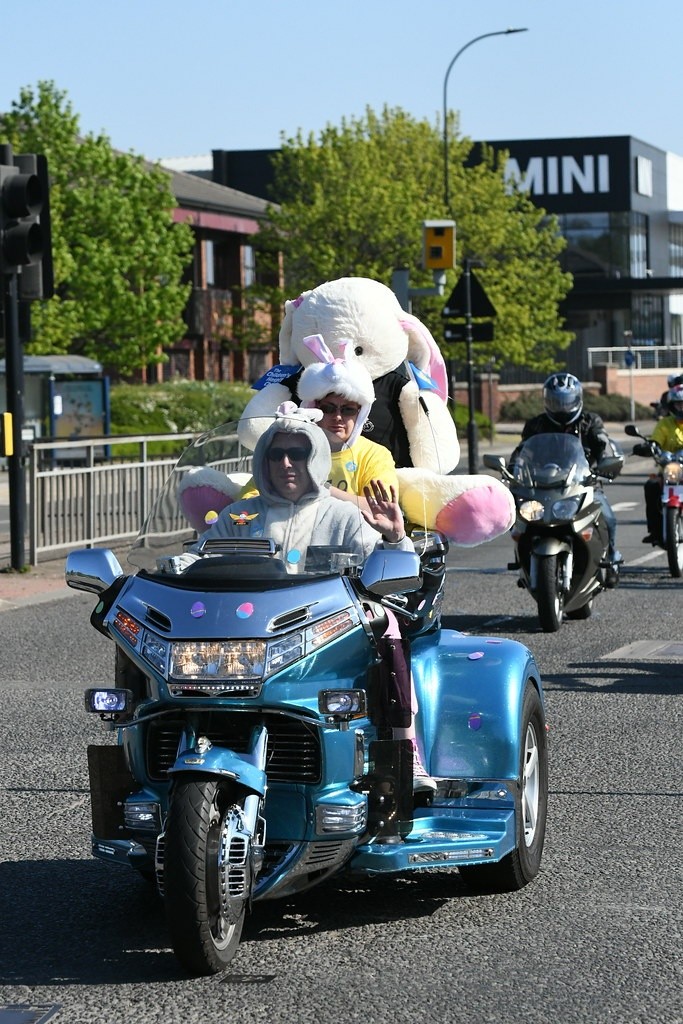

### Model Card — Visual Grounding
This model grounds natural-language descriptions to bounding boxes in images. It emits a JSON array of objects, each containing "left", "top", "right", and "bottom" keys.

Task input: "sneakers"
[
  {"left": 640, "top": 531, "right": 661, "bottom": 543},
  {"left": 604, "top": 563, "right": 621, "bottom": 587},
  {"left": 394, "top": 735, "right": 438, "bottom": 793}
]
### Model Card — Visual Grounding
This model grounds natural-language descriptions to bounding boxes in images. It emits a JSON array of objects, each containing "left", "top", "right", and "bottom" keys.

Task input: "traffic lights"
[{"left": 0, "top": 164, "right": 43, "bottom": 278}]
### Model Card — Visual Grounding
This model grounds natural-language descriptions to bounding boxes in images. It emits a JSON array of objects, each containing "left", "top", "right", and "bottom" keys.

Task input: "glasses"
[
  {"left": 266, "top": 446, "right": 308, "bottom": 461},
  {"left": 318, "top": 401, "right": 361, "bottom": 416}
]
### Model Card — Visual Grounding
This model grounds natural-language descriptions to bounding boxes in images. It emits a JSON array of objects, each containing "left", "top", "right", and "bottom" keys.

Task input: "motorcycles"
[
  {"left": 58, "top": 415, "right": 552, "bottom": 980},
  {"left": 624, "top": 422, "right": 683, "bottom": 579},
  {"left": 483, "top": 432, "right": 625, "bottom": 633}
]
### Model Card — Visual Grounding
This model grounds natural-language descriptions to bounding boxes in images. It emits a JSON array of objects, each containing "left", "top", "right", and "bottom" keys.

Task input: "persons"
[
  {"left": 229, "top": 362, "right": 400, "bottom": 519},
  {"left": 631, "top": 385, "right": 683, "bottom": 545},
  {"left": 183, "top": 401, "right": 438, "bottom": 805},
  {"left": 507, "top": 372, "right": 625, "bottom": 592}
]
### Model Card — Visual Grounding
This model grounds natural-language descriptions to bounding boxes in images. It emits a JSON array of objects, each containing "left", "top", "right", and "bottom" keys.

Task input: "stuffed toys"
[{"left": 177, "top": 277, "right": 517, "bottom": 551}]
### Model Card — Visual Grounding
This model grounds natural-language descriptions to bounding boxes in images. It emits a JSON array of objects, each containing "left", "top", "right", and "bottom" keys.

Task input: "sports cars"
[{"left": 649, "top": 400, "right": 672, "bottom": 422}]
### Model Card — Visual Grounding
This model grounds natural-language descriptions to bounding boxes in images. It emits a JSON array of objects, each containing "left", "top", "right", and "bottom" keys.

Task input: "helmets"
[
  {"left": 666, "top": 371, "right": 679, "bottom": 385},
  {"left": 668, "top": 384, "right": 683, "bottom": 420},
  {"left": 543, "top": 371, "right": 583, "bottom": 426}
]
